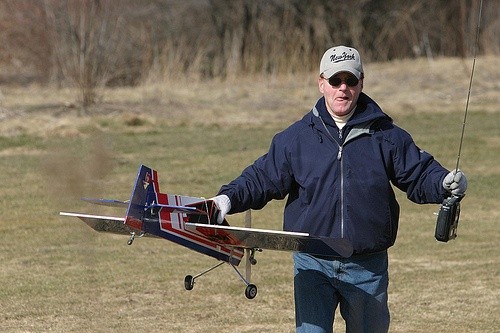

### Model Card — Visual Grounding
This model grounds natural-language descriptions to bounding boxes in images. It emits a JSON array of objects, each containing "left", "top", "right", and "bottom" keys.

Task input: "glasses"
[{"left": 319, "top": 75, "right": 364, "bottom": 85}]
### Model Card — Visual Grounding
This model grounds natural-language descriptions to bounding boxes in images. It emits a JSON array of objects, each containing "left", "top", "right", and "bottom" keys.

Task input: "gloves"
[
  {"left": 206, "top": 194, "right": 231, "bottom": 224},
  {"left": 442, "top": 168, "right": 467, "bottom": 196}
]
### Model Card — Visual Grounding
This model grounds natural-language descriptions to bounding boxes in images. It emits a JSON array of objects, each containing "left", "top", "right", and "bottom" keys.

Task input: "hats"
[{"left": 319, "top": 45, "right": 364, "bottom": 80}]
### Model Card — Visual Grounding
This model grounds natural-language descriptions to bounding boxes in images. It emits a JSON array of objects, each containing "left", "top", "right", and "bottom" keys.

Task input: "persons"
[{"left": 204, "top": 42, "right": 469, "bottom": 333}]
